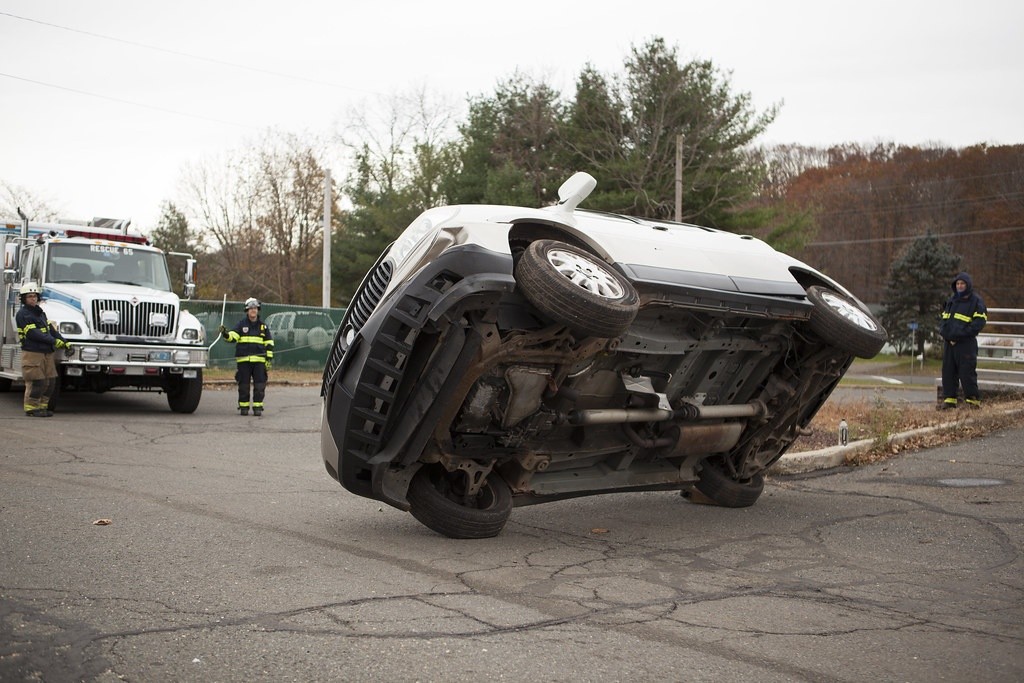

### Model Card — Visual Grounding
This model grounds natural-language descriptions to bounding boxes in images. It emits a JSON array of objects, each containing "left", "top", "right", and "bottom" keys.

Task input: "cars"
[{"left": 320, "top": 171, "right": 888, "bottom": 539}]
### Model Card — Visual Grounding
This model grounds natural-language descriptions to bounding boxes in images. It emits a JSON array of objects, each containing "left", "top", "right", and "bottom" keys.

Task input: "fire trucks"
[{"left": 0, "top": 207, "right": 227, "bottom": 414}]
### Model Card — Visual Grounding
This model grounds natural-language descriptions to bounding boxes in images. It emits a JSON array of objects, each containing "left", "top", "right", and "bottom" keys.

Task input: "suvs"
[{"left": 188, "top": 311, "right": 340, "bottom": 368}]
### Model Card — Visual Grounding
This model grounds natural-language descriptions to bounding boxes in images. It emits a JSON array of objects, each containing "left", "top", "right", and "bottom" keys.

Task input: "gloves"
[
  {"left": 218, "top": 325, "right": 227, "bottom": 337},
  {"left": 53, "top": 338, "right": 66, "bottom": 350},
  {"left": 265, "top": 361, "right": 273, "bottom": 371},
  {"left": 64, "top": 342, "right": 71, "bottom": 350}
]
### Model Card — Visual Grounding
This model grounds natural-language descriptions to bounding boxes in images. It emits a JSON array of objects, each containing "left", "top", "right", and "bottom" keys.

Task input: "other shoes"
[
  {"left": 254, "top": 408, "right": 261, "bottom": 416},
  {"left": 26, "top": 408, "right": 54, "bottom": 417},
  {"left": 241, "top": 407, "right": 248, "bottom": 415},
  {"left": 966, "top": 402, "right": 980, "bottom": 410},
  {"left": 940, "top": 403, "right": 956, "bottom": 411}
]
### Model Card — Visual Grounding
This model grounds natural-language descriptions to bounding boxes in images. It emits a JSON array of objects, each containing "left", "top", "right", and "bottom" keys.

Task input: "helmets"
[
  {"left": 19, "top": 282, "right": 39, "bottom": 295},
  {"left": 244, "top": 297, "right": 260, "bottom": 311}
]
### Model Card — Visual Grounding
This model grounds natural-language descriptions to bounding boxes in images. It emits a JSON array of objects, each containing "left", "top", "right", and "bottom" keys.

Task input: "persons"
[
  {"left": 219, "top": 297, "right": 274, "bottom": 415},
  {"left": 15, "top": 282, "right": 71, "bottom": 416},
  {"left": 936, "top": 272, "right": 987, "bottom": 410}
]
